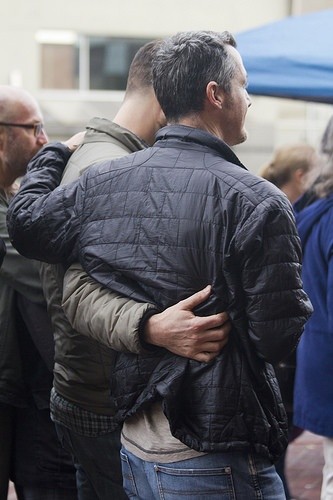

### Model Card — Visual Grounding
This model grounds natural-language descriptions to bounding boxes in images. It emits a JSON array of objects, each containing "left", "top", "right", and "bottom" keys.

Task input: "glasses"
[{"left": 1, "top": 121, "right": 45, "bottom": 137}]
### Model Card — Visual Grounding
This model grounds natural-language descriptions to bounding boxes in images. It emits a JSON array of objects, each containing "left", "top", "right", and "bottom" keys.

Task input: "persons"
[
  {"left": 0, "top": 84, "right": 82, "bottom": 499},
  {"left": 7, "top": 30, "right": 314, "bottom": 499},
  {"left": 258, "top": 141, "right": 321, "bottom": 208},
  {"left": 291, "top": 112, "right": 333, "bottom": 500},
  {"left": 34, "top": 36, "right": 230, "bottom": 498}
]
